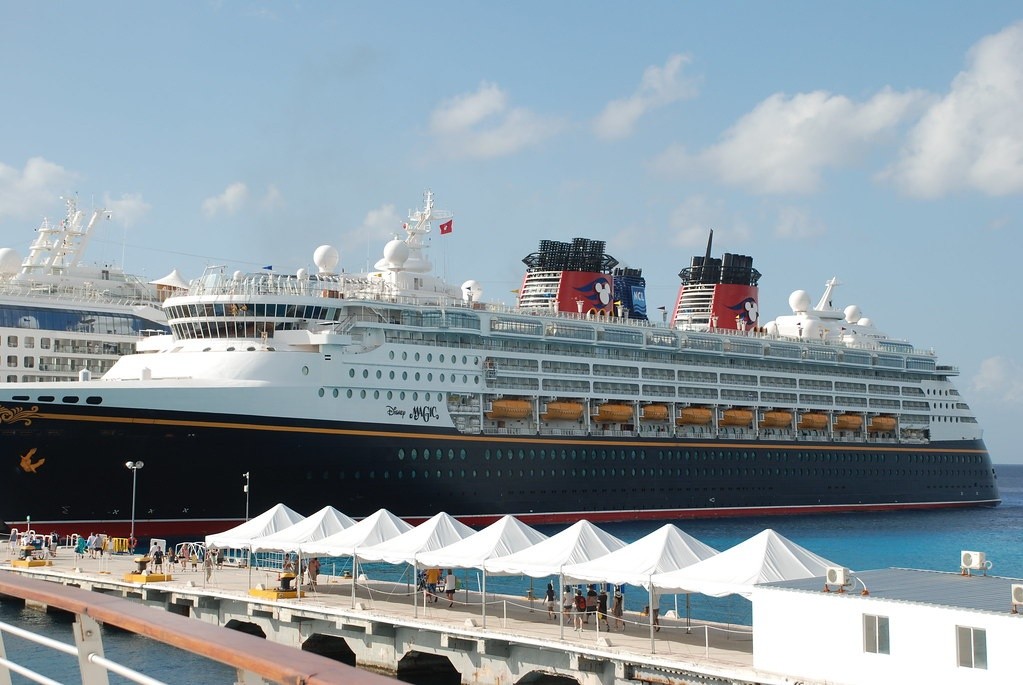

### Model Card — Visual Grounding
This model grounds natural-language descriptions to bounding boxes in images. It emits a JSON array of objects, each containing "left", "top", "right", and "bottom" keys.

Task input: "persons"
[
  {"left": 443, "top": 569, "right": 455, "bottom": 608},
  {"left": 148, "top": 542, "right": 223, "bottom": 583},
  {"left": 26, "top": 530, "right": 30, "bottom": 542},
  {"left": 75, "top": 535, "right": 86, "bottom": 559},
  {"left": 389, "top": 315, "right": 395, "bottom": 324},
  {"left": 107, "top": 536, "right": 114, "bottom": 560},
  {"left": 542, "top": 583, "right": 661, "bottom": 632},
  {"left": 657, "top": 426, "right": 660, "bottom": 432},
  {"left": 283, "top": 552, "right": 293, "bottom": 572},
  {"left": 426, "top": 569, "right": 441, "bottom": 603},
  {"left": 29, "top": 535, "right": 34, "bottom": 545},
  {"left": 293, "top": 554, "right": 304, "bottom": 589},
  {"left": 87, "top": 532, "right": 103, "bottom": 559},
  {"left": 8, "top": 530, "right": 18, "bottom": 555},
  {"left": 128, "top": 533, "right": 137, "bottom": 555},
  {"left": 48, "top": 530, "right": 59, "bottom": 557},
  {"left": 94, "top": 344, "right": 100, "bottom": 354},
  {"left": 307, "top": 557, "right": 321, "bottom": 586}
]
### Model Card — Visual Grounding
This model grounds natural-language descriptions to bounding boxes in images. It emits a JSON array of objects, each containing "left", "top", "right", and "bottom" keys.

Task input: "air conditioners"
[
  {"left": 826, "top": 567, "right": 851, "bottom": 586},
  {"left": 1011, "top": 584, "right": 1023, "bottom": 606},
  {"left": 960, "top": 551, "right": 986, "bottom": 570}
]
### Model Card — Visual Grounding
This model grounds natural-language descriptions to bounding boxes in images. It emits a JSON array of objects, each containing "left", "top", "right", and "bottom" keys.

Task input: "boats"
[
  {"left": 797, "top": 413, "right": 828, "bottom": 429},
  {"left": 759, "top": 410, "right": 793, "bottom": 429},
  {"left": 868, "top": 417, "right": 897, "bottom": 432},
  {"left": 484, "top": 399, "right": 533, "bottom": 421},
  {"left": 675, "top": 408, "right": 713, "bottom": 425},
  {"left": 640, "top": 403, "right": 672, "bottom": 422},
  {"left": 833, "top": 414, "right": 863, "bottom": 429},
  {"left": 719, "top": 408, "right": 757, "bottom": 427},
  {"left": 539, "top": 401, "right": 585, "bottom": 423},
  {"left": 591, "top": 404, "right": 634, "bottom": 422}
]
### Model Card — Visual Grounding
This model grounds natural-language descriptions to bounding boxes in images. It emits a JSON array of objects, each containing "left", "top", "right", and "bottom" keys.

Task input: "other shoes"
[
  {"left": 656, "top": 627, "right": 661, "bottom": 632},
  {"left": 566, "top": 618, "right": 571, "bottom": 623},
  {"left": 435, "top": 597, "right": 437, "bottom": 601},
  {"left": 553, "top": 615, "right": 556, "bottom": 620},
  {"left": 427, "top": 600, "right": 432, "bottom": 603},
  {"left": 449, "top": 602, "right": 454, "bottom": 607}
]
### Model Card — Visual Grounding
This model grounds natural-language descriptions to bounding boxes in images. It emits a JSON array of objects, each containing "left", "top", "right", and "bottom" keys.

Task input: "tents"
[
  {"left": 296, "top": 508, "right": 417, "bottom": 602},
  {"left": 649, "top": 529, "right": 856, "bottom": 655},
  {"left": 351, "top": 512, "right": 479, "bottom": 612},
  {"left": 481, "top": 520, "right": 630, "bottom": 630},
  {"left": 248, "top": 506, "right": 358, "bottom": 590},
  {"left": 559, "top": 523, "right": 722, "bottom": 641},
  {"left": 414, "top": 513, "right": 551, "bottom": 619},
  {"left": 204, "top": 503, "right": 307, "bottom": 586}
]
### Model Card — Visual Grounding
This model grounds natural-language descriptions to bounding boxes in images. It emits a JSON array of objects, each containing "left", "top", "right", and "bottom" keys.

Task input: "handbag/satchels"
[
  {"left": 73, "top": 545, "right": 80, "bottom": 552},
  {"left": 598, "top": 613, "right": 603, "bottom": 620},
  {"left": 456, "top": 579, "right": 462, "bottom": 589}
]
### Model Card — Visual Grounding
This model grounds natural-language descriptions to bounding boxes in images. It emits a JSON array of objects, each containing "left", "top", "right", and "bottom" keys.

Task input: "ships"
[
  {"left": 1, "top": 193, "right": 1003, "bottom": 543},
  {"left": 2, "top": 197, "right": 186, "bottom": 382}
]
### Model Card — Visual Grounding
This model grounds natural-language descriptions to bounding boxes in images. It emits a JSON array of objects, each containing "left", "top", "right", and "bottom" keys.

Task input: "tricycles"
[{"left": 416, "top": 570, "right": 446, "bottom": 593}]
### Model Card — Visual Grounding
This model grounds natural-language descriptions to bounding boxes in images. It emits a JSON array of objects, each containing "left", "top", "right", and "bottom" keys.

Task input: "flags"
[{"left": 439, "top": 219, "right": 452, "bottom": 234}]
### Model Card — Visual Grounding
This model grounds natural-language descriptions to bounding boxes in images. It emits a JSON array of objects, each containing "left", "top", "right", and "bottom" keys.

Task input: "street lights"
[
  {"left": 242, "top": 471, "right": 252, "bottom": 522},
  {"left": 124, "top": 461, "right": 145, "bottom": 554}
]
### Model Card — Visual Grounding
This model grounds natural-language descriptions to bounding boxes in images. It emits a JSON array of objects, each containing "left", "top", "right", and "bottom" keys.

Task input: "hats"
[
  {"left": 615, "top": 591, "right": 622, "bottom": 598},
  {"left": 600, "top": 589, "right": 605, "bottom": 595}
]
